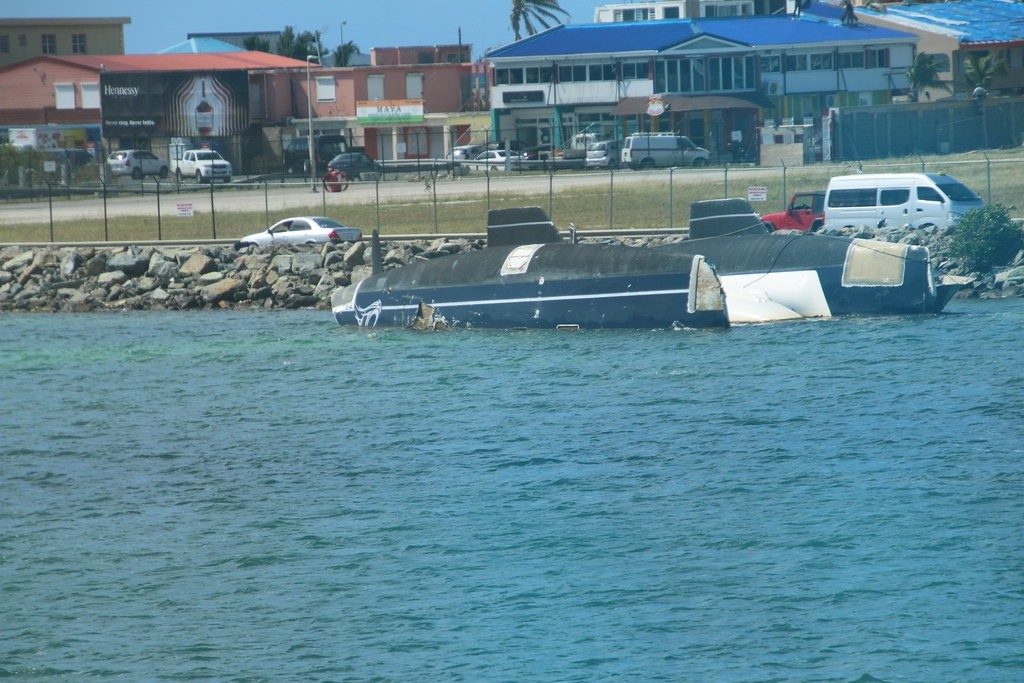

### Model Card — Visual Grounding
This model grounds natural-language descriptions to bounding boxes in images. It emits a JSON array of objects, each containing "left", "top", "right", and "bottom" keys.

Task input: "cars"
[
  {"left": 326, "top": 152, "right": 384, "bottom": 178},
  {"left": 238, "top": 216, "right": 362, "bottom": 248},
  {"left": 473, "top": 150, "right": 523, "bottom": 171}
]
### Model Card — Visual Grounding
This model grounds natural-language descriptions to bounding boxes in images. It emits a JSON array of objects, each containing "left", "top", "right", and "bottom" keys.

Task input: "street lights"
[
  {"left": 340, "top": 21, "right": 346, "bottom": 66},
  {"left": 306, "top": 55, "right": 318, "bottom": 192}
]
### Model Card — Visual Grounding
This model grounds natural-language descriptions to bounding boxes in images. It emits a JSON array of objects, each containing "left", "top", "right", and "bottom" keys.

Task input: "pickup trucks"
[{"left": 172, "top": 149, "right": 232, "bottom": 184}]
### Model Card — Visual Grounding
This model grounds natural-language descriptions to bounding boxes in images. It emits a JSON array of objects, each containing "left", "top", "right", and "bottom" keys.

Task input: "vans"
[
  {"left": 571, "top": 133, "right": 604, "bottom": 149},
  {"left": 282, "top": 134, "right": 348, "bottom": 173},
  {"left": 621, "top": 130, "right": 711, "bottom": 170},
  {"left": 585, "top": 140, "right": 624, "bottom": 168},
  {"left": 447, "top": 146, "right": 480, "bottom": 160},
  {"left": 823, "top": 172, "right": 985, "bottom": 232}
]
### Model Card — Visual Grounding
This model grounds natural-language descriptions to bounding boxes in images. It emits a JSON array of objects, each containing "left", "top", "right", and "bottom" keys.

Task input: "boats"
[{"left": 332, "top": 197, "right": 978, "bottom": 332}]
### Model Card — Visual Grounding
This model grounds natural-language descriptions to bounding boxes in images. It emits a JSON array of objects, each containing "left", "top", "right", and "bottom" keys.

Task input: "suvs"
[
  {"left": 40, "top": 149, "right": 96, "bottom": 181},
  {"left": 759, "top": 190, "right": 827, "bottom": 233},
  {"left": 108, "top": 149, "right": 168, "bottom": 179}
]
